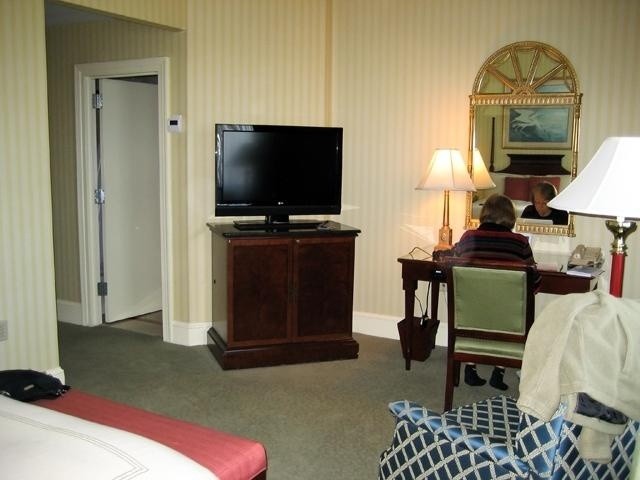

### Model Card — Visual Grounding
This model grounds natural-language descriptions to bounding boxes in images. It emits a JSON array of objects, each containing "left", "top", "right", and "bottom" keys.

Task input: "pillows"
[
  {"left": 529, "top": 177, "right": 559, "bottom": 203},
  {"left": 505, "top": 177, "right": 529, "bottom": 201}
]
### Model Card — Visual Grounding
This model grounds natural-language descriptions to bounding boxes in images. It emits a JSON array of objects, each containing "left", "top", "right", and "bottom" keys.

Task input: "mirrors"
[{"left": 464, "top": 40, "right": 581, "bottom": 237}]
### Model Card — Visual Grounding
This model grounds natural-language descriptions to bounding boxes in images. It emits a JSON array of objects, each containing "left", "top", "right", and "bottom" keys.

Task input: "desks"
[{"left": 397, "top": 244, "right": 605, "bottom": 371}]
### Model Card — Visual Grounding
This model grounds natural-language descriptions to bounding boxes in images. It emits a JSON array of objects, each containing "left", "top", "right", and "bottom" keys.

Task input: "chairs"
[
  {"left": 378, "top": 293, "right": 639, "bottom": 480},
  {"left": 440, "top": 255, "right": 534, "bottom": 411}
]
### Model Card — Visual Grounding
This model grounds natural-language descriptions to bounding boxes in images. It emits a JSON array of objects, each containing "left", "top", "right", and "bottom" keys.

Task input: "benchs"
[{"left": 0, "top": 380, "right": 268, "bottom": 479}]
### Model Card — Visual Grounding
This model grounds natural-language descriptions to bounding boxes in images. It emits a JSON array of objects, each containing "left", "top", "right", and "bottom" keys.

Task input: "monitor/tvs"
[{"left": 215, "top": 124, "right": 343, "bottom": 228}]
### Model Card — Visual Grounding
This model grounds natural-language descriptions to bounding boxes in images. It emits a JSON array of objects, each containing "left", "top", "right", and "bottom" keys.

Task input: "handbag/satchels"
[{"left": 0, "top": 369, "right": 72, "bottom": 403}]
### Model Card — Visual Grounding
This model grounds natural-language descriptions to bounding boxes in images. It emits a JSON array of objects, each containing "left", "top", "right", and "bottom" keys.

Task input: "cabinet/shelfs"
[{"left": 205, "top": 219, "right": 362, "bottom": 370}]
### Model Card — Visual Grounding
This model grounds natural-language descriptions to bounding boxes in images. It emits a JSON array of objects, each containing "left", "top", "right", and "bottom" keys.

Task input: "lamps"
[
  {"left": 414, "top": 148, "right": 477, "bottom": 261},
  {"left": 545, "top": 137, "right": 640, "bottom": 300},
  {"left": 472, "top": 147, "right": 495, "bottom": 190}
]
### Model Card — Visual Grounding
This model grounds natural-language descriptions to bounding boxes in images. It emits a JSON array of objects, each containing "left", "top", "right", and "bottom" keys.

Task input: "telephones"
[{"left": 567, "top": 244, "right": 602, "bottom": 266}]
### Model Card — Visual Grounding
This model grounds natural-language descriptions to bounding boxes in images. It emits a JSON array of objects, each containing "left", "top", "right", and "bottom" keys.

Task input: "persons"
[
  {"left": 451, "top": 192, "right": 540, "bottom": 391},
  {"left": 520, "top": 182, "right": 568, "bottom": 225}
]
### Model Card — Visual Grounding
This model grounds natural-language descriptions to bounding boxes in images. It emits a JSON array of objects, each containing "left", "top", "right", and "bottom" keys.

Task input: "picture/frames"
[{"left": 501, "top": 78, "right": 575, "bottom": 150}]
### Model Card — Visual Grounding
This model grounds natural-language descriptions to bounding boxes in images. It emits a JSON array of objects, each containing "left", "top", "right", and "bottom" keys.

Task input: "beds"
[
  {"left": 470, "top": 117, "right": 572, "bottom": 220},
  {"left": 1, "top": 398, "right": 217, "bottom": 480}
]
